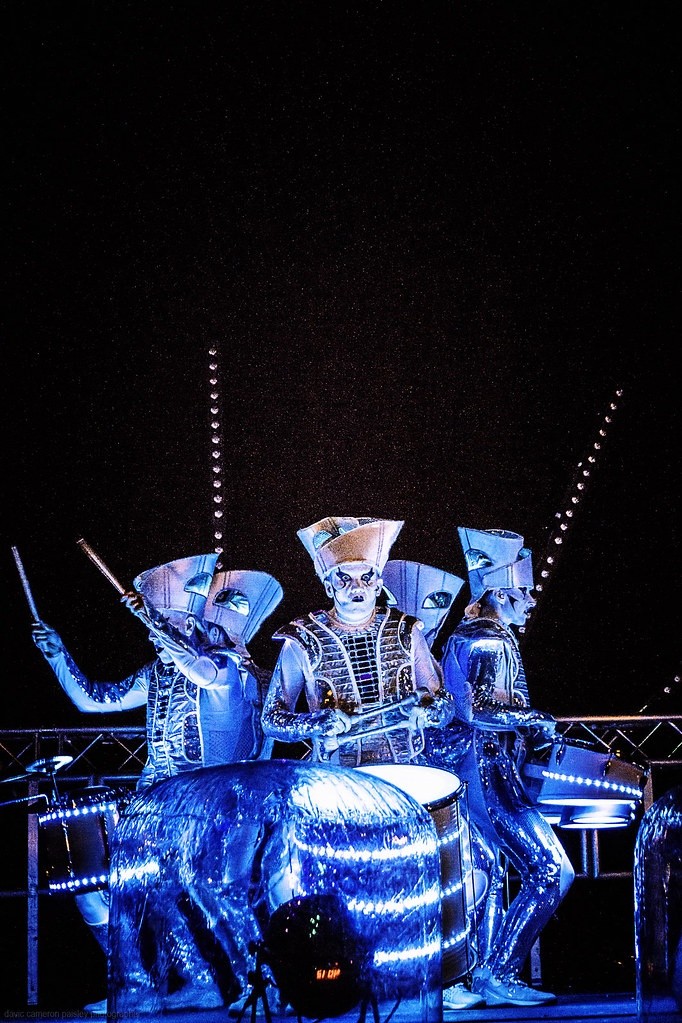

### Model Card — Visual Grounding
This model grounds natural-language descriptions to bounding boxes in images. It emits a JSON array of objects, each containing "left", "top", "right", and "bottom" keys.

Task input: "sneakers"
[
  {"left": 161, "top": 981, "right": 224, "bottom": 1010},
  {"left": 473, "top": 969, "right": 555, "bottom": 1006},
  {"left": 442, "top": 983, "right": 487, "bottom": 1009},
  {"left": 83, "top": 990, "right": 163, "bottom": 1016},
  {"left": 228, "top": 986, "right": 292, "bottom": 1017}
]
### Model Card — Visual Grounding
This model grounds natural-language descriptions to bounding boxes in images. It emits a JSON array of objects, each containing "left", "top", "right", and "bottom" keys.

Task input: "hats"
[
  {"left": 298, "top": 517, "right": 404, "bottom": 578},
  {"left": 135, "top": 553, "right": 218, "bottom": 621},
  {"left": 456, "top": 527, "right": 535, "bottom": 603},
  {"left": 379, "top": 560, "right": 465, "bottom": 642},
  {"left": 201, "top": 570, "right": 284, "bottom": 643}
]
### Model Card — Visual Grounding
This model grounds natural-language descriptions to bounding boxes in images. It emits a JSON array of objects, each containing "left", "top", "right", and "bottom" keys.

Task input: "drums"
[
  {"left": 351, "top": 759, "right": 477, "bottom": 988},
  {"left": 35, "top": 794, "right": 134, "bottom": 896},
  {"left": 522, "top": 739, "right": 649, "bottom": 831}
]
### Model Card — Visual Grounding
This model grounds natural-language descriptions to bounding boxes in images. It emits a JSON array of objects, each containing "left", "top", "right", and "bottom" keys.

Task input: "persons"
[{"left": 32, "top": 517, "right": 576, "bottom": 1015}]
